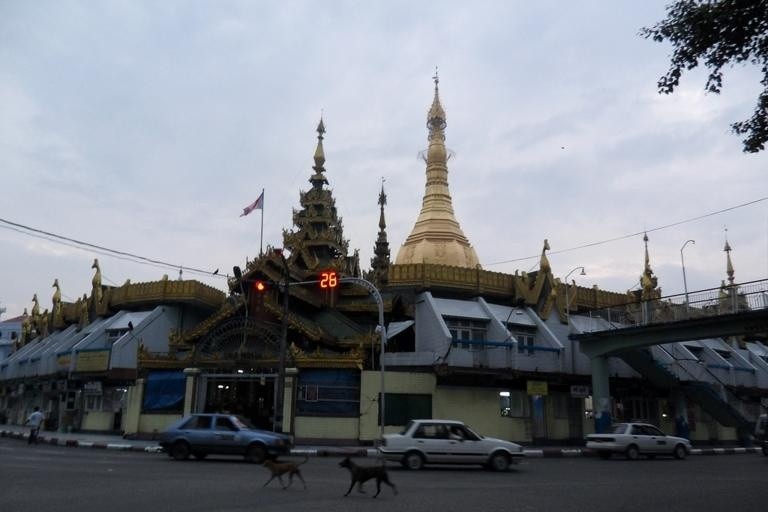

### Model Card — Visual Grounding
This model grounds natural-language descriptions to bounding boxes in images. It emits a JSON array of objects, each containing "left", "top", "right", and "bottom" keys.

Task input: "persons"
[
  {"left": 24, "top": 406, "right": 45, "bottom": 443},
  {"left": 449, "top": 426, "right": 463, "bottom": 440}
]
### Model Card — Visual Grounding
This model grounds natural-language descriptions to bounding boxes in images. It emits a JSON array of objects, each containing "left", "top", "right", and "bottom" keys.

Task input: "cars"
[
  {"left": 159, "top": 412, "right": 296, "bottom": 465},
  {"left": 377, "top": 417, "right": 524, "bottom": 470},
  {"left": 585, "top": 421, "right": 691, "bottom": 461}
]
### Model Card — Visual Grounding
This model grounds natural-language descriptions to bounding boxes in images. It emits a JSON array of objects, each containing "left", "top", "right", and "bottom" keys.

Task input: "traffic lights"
[
  {"left": 254, "top": 281, "right": 270, "bottom": 294},
  {"left": 318, "top": 270, "right": 339, "bottom": 290}
]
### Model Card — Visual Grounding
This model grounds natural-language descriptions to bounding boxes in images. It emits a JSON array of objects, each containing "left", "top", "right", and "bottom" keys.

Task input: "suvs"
[{"left": 754, "top": 414, "right": 768, "bottom": 455}]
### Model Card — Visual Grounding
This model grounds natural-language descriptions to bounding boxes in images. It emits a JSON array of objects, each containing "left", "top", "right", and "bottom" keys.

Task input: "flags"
[{"left": 239, "top": 191, "right": 262, "bottom": 217}]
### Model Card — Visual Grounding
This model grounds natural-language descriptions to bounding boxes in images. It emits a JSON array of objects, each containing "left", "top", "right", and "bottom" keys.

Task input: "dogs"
[
  {"left": 338, "top": 455, "right": 400, "bottom": 498},
  {"left": 262, "top": 454, "right": 312, "bottom": 492}
]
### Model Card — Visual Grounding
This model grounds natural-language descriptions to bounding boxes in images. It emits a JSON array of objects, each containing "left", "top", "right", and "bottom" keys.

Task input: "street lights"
[
  {"left": 505, "top": 306, "right": 526, "bottom": 349},
  {"left": 680, "top": 239, "right": 696, "bottom": 318},
  {"left": 268, "top": 246, "right": 291, "bottom": 431},
  {"left": 564, "top": 265, "right": 586, "bottom": 336},
  {"left": 232, "top": 264, "right": 249, "bottom": 346}
]
